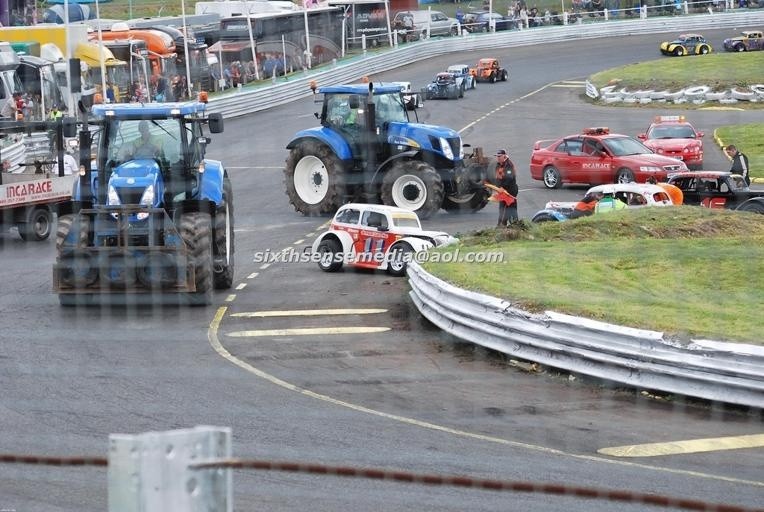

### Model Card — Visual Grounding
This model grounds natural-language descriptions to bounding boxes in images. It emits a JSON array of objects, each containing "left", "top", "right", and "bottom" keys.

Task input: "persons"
[
  {"left": 106, "top": 82, "right": 113, "bottom": 101},
  {"left": 726, "top": 145, "right": 750, "bottom": 189},
  {"left": 135, "top": 89, "right": 147, "bottom": 103},
  {"left": 403, "top": 10, "right": 414, "bottom": 43},
  {"left": 16, "top": 93, "right": 26, "bottom": 112},
  {"left": 494, "top": 149, "right": 518, "bottom": 227},
  {"left": 1, "top": 92, "right": 21, "bottom": 118},
  {"left": 110, "top": 82, "right": 120, "bottom": 102},
  {"left": 48, "top": 103, "right": 62, "bottom": 121},
  {"left": 224, "top": 65, "right": 231, "bottom": 79},
  {"left": 262, "top": 51, "right": 274, "bottom": 78},
  {"left": 644, "top": 175, "right": 657, "bottom": 185},
  {"left": 52, "top": 148, "right": 79, "bottom": 175},
  {"left": 229, "top": 62, "right": 241, "bottom": 88},
  {"left": 133, "top": 121, "right": 162, "bottom": 158}
]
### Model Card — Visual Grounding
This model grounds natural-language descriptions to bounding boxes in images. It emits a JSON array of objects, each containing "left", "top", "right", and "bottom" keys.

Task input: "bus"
[
  {"left": 131, "top": 25, "right": 211, "bottom": 90},
  {"left": 208, "top": 7, "right": 342, "bottom": 83},
  {"left": 91, "top": 30, "right": 180, "bottom": 98},
  {"left": 98, "top": 38, "right": 154, "bottom": 102},
  {"left": 17, "top": 55, "right": 68, "bottom": 119},
  {"left": 0, "top": 41, "right": 28, "bottom": 117}
]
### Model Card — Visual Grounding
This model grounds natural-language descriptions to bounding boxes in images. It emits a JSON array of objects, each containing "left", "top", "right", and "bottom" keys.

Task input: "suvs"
[
  {"left": 465, "top": 10, "right": 514, "bottom": 32},
  {"left": 394, "top": 10, "right": 459, "bottom": 42}
]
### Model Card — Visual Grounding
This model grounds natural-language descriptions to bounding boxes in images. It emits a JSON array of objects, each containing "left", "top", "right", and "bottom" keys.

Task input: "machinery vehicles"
[
  {"left": 282, "top": 77, "right": 493, "bottom": 218},
  {"left": 52, "top": 90, "right": 235, "bottom": 307}
]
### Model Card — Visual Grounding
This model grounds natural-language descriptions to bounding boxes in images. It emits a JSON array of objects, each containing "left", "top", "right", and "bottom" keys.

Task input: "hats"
[
  {"left": 52, "top": 103, "right": 58, "bottom": 108},
  {"left": 494, "top": 150, "right": 505, "bottom": 157}
]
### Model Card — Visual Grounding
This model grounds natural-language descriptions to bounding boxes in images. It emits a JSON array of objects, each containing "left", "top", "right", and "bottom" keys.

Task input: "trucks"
[
  {"left": 0, "top": 24, "right": 128, "bottom": 103},
  {"left": 194, "top": 1, "right": 297, "bottom": 16},
  {"left": 12, "top": 42, "right": 97, "bottom": 110},
  {"left": 0, "top": 172, "right": 75, "bottom": 242}
]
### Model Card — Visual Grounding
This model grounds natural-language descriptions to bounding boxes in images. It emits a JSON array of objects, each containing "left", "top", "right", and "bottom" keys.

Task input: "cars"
[
  {"left": 469, "top": 58, "right": 508, "bottom": 82},
  {"left": 303, "top": 201, "right": 457, "bottom": 277},
  {"left": 420, "top": 71, "right": 465, "bottom": 100},
  {"left": 532, "top": 183, "right": 672, "bottom": 223},
  {"left": 392, "top": 81, "right": 419, "bottom": 109},
  {"left": 447, "top": 64, "right": 476, "bottom": 91},
  {"left": 723, "top": 30, "right": 764, "bottom": 52},
  {"left": 667, "top": 170, "right": 764, "bottom": 214},
  {"left": 530, "top": 127, "right": 690, "bottom": 190},
  {"left": 638, "top": 114, "right": 705, "bottom": 170},
  {"left": 660, "top": 33, "right": 712, "bottom": 55}
]
